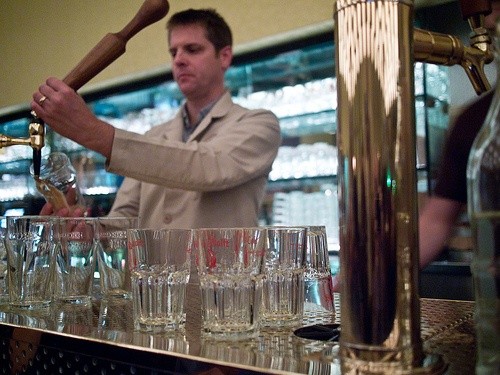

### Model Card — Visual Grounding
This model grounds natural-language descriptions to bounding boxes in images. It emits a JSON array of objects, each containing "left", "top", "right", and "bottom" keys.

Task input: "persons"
[
  {"left": 329, "top": 0, "right": 500, "bottom": 296},
  {"left": 31, "top": 9, "right": 280, "bottom": 269}
]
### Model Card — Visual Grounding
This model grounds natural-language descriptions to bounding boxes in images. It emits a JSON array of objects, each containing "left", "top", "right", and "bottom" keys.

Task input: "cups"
[
  {"left": 126, "top": 228, "right": 193, "bottom": 333},
  {"left": 0, "top": 295, "right": 333, "bottom": 375},
  {"left": 1, "top": 215, "right": 57, "bottom": 308},
  {"left": 91, "top": 217, "right": 138, "bottom": 300},
  {"left": 193, "top": 228, "right": 263, "bottom": 340},
  {"left": 277, "top": 225, "right": 335, "bottom": 317},
  {"left": 52, "top": 218, "right": 98, "bottom": 303},
  {"left": 28, "top": 151, "right": 86, "bottom": 217},
  {"left": 258, "top": 226, "right": 308, "bottom": 326}
]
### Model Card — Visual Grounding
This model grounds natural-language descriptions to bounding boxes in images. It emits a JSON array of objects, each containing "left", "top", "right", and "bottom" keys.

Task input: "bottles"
[{"left": 465, "top": 81, "right": 500, "bottom": 374}]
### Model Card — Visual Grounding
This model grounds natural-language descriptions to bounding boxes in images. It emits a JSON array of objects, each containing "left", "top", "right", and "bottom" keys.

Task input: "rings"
[{"left": 39, "top": 96, "right": 46, "bottom": 105}]
